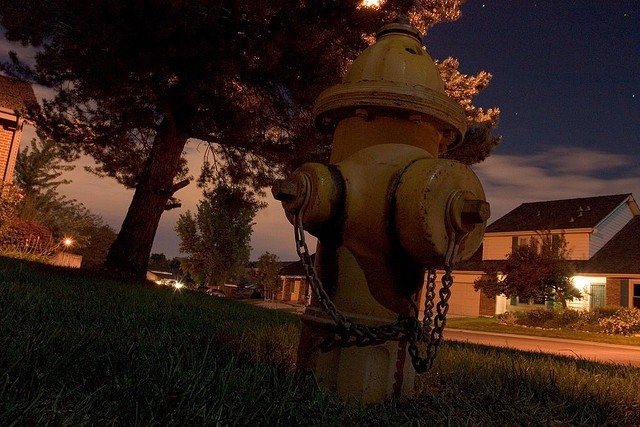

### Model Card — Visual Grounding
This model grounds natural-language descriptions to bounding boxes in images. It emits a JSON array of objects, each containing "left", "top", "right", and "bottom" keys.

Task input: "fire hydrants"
[{"left": 272, "top": 23, "right": 491, "bottom": 409}]
